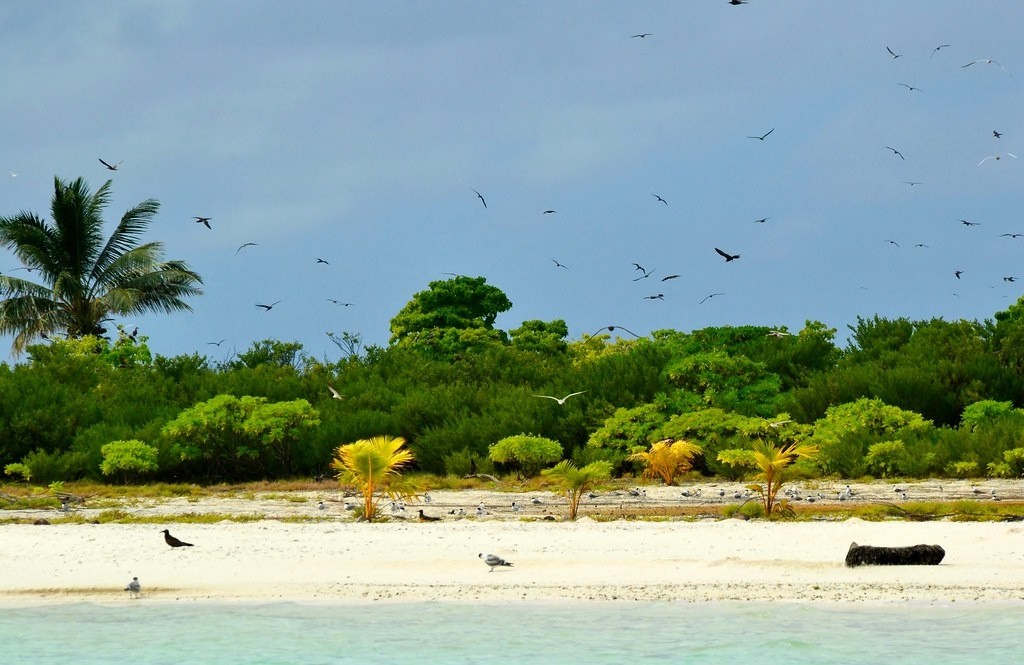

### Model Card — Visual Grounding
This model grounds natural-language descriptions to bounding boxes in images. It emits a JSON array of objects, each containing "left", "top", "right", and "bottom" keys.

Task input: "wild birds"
[
  {"left": 160, "top": 528, "right": 195, "bottom": 550},
  {"left": 630, "top": 32, "right": 653, "bottom": 39},
  {"left": 586, "top": 326, "right": 643, "bottom": 343},
  {"left": 236, "top": 243, "right": 257, "bottom": 255},
  {"left": 98, "top": 157, "right": 124, "bottom": 172},
  {"left": 883, "top": 42, "right": 1023, "bottom": 299},
  {"left": 7, "top": 267, "right": 43, "bottom": 272},
  {"left": 311, "top": 479, "right": 1000, "bottom": 523},
  {"left": 124, "top": 577, "right": 140, "bottom": 599},
  {"left": 727, "top": 0, "right": 748, "bottom": 6},
  {"left": 478, "top": 552, "right": 513, "bottom": 572},
  {"left": 192, "top": 216, "right": 214, "bottom": 230},
  {"left": 38, "top": 126, "right": 776, "bottom": 338},
  {"left": 326, "top": 385, "right": 345, "bottom": 401},
  {"left": 532, "top": 389, "right": 589, "bottom": 405}
]
